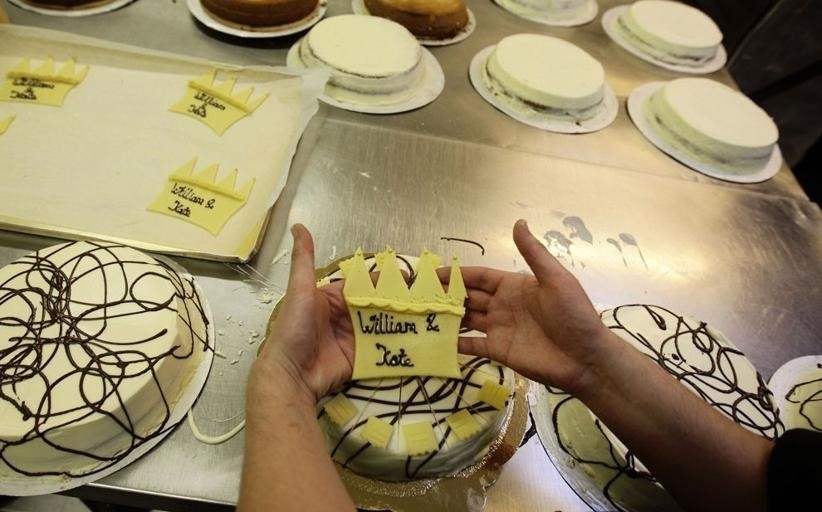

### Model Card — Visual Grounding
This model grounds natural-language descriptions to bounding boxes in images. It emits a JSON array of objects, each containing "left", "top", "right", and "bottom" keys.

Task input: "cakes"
[
  {"left": 470, "top": 34, "right": 619, "bottom": 134},
  {"left": 543, "top": 302, "right": 775, "bottom": 511},
  {"left": 11, "top": 1, "right": 132, "bottom": 16},
  {"left": 783, "top": 363, "right": 822, "bottom": 429},
  {"left": 351, "top": 1, "right": 477, "bottom": 46},
  {"left": 0, "top": 239, "right": 211, "bottom": 482},
  {"left": 271, "top": 252, "right": 517, "bottom": 483},
  {"left": 627, "top": 77, "right": 781, "bottom": 183},
  {"left": 602, "top": 0, "right": 727, "bottom": 74},
  {"left": 286, "top": 14, "right": 445, "bottom": 115},
  {"left": 187, "top": 0, "right": 327, "bottom": 38}
]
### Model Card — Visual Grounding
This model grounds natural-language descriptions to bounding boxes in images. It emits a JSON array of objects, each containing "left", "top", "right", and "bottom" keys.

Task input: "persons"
[{"left": 233, "top": 219, "right": 822, "bottom": 512}]
